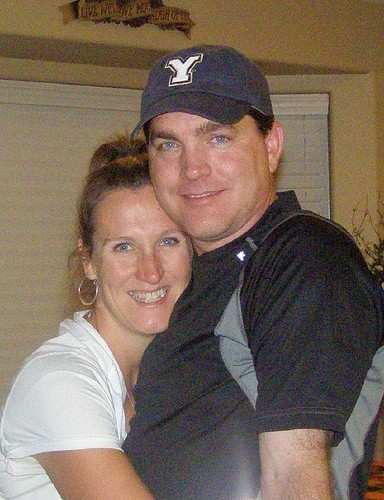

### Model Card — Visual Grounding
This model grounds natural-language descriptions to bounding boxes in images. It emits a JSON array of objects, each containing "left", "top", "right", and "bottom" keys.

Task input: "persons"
[
  {"left": 0, "top": 138, "right": 194, "bottom": 500},
  {"left": 121, "top": 43, "right": 384, "bottom": 500}
]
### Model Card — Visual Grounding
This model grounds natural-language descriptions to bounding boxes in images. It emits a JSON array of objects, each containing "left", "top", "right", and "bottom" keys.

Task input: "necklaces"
[{"left": 87, "top": 309, "right": 136, "bottom": 415}]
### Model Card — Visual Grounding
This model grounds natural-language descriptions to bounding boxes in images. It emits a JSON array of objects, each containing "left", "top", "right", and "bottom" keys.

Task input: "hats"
[{"left": 130, "top": 45, "right": 273, "bottom": 142}]
